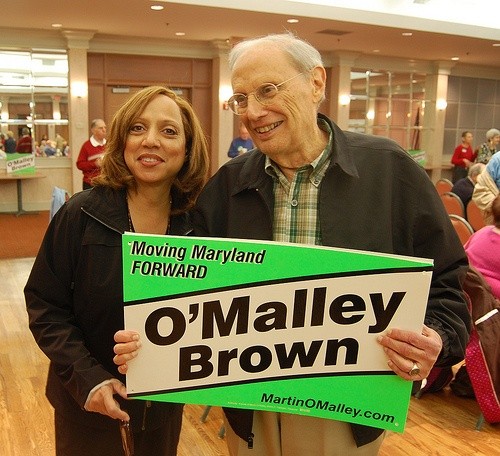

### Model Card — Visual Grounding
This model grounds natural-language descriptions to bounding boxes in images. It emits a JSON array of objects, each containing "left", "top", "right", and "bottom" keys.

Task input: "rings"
[{"left": 408, "top": 362, "right": 420, "bottom": 376}]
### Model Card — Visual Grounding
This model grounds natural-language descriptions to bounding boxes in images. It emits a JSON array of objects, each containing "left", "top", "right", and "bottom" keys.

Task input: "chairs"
[
  {"left": 465, "top": 268, "right": 500, "bottom": 399},
  {"left": 440, "top": 192, "right": 465, "bottom": 222},
  {"left": 448, "top": 214, "right": 474, "bottom": 249},
  {"left": 467, "top": 199, "right": 486, "bottom": 233},
  {"left": 436, "top": 178, "right": 454, "bottom": 198}
]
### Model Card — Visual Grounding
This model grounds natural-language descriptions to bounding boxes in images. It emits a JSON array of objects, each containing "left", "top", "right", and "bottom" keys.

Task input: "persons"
[
  {"left": 228, "top": 126, "right": 254, "bottom": 159},
  {"left": 475, "top": 128, "right": 500, "bottom": 169},
  {"left": 113, "top": 28, "right": 472, "bottom": 456},
  {"left": 0, "top": 127, "right": 72, "bottom": 159},
  {"left": 451, "top": 131, "right": 480, "bottom": 182},
  {"left": 463, "top": 192, "right": 500, "bottom": 303},
  {"left": 75, "top": 117, "right": 111, "bottom": 191},
  {"left": 475, "top": 150, "right": 500, "bottom": 227},
  {"left": 24, "top": 87, "right": 218, "bottom": 456}
]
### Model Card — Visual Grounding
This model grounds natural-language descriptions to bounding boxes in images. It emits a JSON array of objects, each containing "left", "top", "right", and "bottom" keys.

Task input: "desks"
[{"left": 0, "top": 172, "right": 47, "bottom": 216}]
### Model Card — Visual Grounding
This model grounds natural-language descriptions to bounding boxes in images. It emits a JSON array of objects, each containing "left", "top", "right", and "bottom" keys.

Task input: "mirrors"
[
  {"left": 1, "top": 50, "right": 71, "bottom": 158},
  {"left": 349, "top": 69, "right": 426, "bottom": 153}
]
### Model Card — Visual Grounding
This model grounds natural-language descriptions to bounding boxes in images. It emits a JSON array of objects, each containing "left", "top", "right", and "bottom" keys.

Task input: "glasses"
[{"left": 220, "top": 73, "right": 308, "bottom": 115}]
